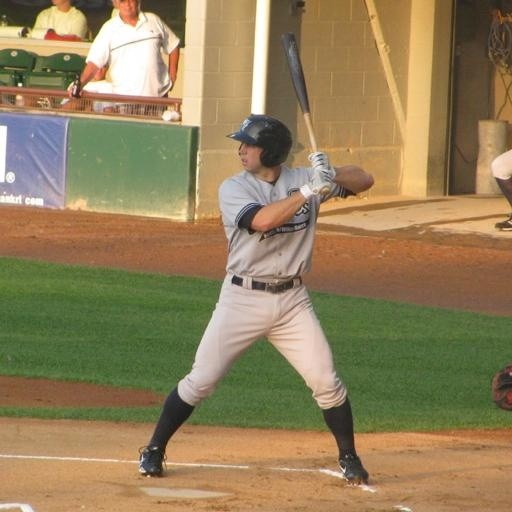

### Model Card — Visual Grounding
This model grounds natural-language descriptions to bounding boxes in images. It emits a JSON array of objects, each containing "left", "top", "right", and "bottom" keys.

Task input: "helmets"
[{"left": 225, "top": 115, "right": 292, "bottom": 168}]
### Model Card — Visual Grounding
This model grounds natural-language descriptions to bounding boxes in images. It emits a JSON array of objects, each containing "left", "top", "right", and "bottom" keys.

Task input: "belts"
[{"left": 232, "top": 275, "right": 303, "bottom": 293}]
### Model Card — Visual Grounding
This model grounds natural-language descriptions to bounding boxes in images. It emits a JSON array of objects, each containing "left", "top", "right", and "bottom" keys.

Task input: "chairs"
[{"left": 0, "top": 49, "right": 87, "bottom": 110}]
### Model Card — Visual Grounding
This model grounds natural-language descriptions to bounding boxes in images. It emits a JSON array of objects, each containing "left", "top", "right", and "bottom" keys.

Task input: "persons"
[
  {"left": 136, "top": 111, "right": 377, "bottom": 486},
  {"left": 489, "top": 148, "right": 512, "bottom": 232},
  {"left": 55, "top": 62, "right": 111, "bottom": 111},
  {"left": 66, "top": 0, "right": 180, "bottom": 116},
  {"left": 28, "top": 1, "right": 91, "bottom": 41}
]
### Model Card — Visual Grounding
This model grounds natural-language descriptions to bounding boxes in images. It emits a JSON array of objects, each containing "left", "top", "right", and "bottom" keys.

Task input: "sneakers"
[
  {"left": 337, "top": 455, "right": 369, "bottom": 484},
  {"left": 138, "top": 445, "right": 168, "bottom": 475},
  {"left": 494, "top": 217, "right": 512, "bottom": 231}
]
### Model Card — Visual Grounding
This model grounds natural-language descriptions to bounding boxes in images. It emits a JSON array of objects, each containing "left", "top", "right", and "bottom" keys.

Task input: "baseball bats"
[{"left": 281, "top": 31, "right": 331, "bottom": 197}]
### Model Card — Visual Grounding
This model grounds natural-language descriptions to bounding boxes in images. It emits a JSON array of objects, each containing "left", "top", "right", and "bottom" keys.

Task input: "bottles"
[{"left": 72, "top": 73, "right": 80, "bottom": 98}]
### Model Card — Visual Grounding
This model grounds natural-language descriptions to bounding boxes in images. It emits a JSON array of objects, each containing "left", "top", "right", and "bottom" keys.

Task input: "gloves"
[
  {"left": 307, "top": 151, "right": 336, "bottom": 183},
  {"left": 299, "top": 167, "right": 333, "bottom": 201}
]
